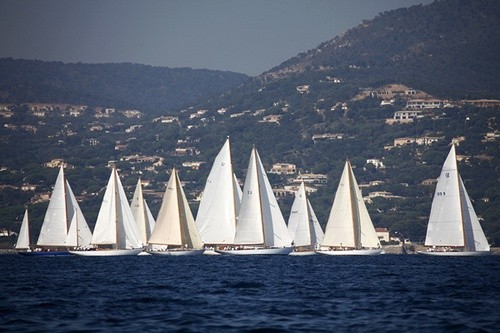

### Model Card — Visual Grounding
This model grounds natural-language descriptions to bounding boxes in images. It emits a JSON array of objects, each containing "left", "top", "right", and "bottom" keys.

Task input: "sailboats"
[
  {"left": 315, "top": 156, "right": 385, "bottom": 255},
  {"left": 13, "top": 135, "right": 327, "bottom": 258},
  {"left": 416, "top": 142, "right": 490, "bottom": 258}
]
